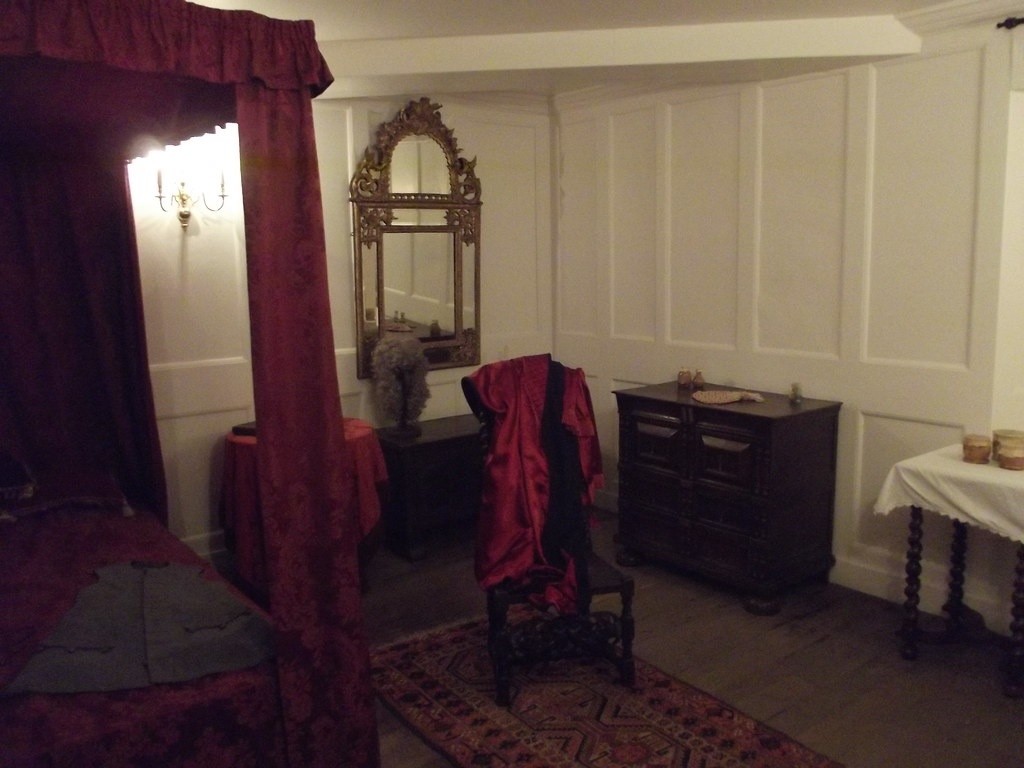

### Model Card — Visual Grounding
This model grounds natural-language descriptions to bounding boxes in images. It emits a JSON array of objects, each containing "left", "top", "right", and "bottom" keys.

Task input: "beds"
[{"left": 0, "top": 0, "right": 385, "bottom": 768}]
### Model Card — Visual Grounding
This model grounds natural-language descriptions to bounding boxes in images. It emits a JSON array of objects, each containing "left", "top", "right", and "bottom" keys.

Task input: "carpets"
[{"left": 368, "top": 602, "right": 849, "bottom": 768}]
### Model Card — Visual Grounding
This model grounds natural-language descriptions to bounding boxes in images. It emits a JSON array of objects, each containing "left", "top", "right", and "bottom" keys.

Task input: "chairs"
[{"left": 461, "top": 354, "right": 640, "bottom": 707}]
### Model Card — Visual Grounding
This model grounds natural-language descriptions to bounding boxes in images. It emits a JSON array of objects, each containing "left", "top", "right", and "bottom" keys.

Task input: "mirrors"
[{"left": 346, "top": 97, "right": 482, "bottom": 380}]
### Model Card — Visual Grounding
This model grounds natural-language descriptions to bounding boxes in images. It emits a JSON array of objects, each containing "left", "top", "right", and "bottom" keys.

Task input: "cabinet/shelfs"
[{"left": 611, "top": 379, "right": 844, "bottom": 615}]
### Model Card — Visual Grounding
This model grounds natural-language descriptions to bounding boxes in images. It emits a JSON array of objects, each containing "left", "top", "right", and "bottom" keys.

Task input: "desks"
[
  {"left": 872, "top": 442, "right": 1024, "bottom": 699},
  {"left": 366, "top": 318, "right": 455, "bottom": 342},
  {"left": 215, "top": 417, "right": 390, "bottom": 595},
  {"left": 379, "top": 411, "right": 483, "bottom": 563}
]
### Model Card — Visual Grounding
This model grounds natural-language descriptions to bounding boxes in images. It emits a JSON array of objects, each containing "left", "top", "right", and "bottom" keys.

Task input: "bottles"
[
  {"left": 692, "top": 369, "right": 704, "bottom": 390},
  {"left": 677, "top": 368, "right": 690, "bottom": 389}
]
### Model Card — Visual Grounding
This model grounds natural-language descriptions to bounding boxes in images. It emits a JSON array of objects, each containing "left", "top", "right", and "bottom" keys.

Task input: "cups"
[{"left": 963, "top": 430, "right": 1024, "bottom": 471}]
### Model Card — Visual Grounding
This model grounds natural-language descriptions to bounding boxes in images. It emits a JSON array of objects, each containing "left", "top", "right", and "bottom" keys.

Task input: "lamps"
[{"left": 154, "top": 165, "right": 227, "bottom": 233}]
